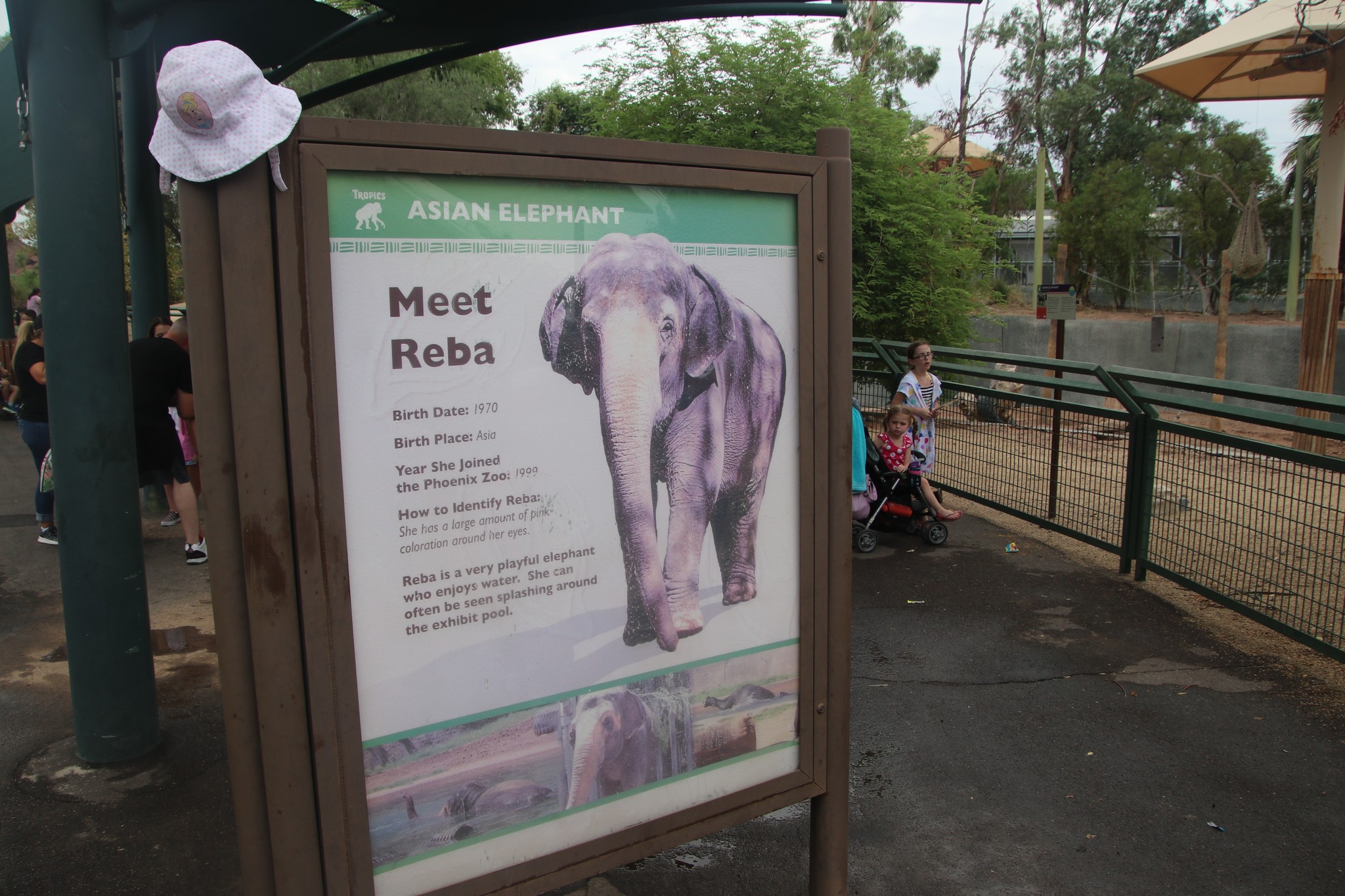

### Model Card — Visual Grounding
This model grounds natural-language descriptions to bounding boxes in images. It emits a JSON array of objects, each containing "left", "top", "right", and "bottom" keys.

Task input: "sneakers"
[
  {"left": 37, "top": 527, "right": 58, "bottom": 545},
  {"left": 3, "top": 405, "right": 18, "bottom": 413}
]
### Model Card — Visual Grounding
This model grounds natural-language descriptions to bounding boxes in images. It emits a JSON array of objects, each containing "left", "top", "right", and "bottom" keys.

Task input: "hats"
[
  {"left": 33, "top": 312, "right": 43, "bottom": 330},
  {"left": 148, "top": 39, "right": 302, "bottom": 196}
]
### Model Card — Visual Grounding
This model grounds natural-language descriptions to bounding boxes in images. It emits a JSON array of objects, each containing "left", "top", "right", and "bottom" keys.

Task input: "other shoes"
[
  {"left": 161, "top": 510, "right": 180, "bottom": 526},
  {"left": 183, "top": 540, "right": 207, "bottom": 564}
]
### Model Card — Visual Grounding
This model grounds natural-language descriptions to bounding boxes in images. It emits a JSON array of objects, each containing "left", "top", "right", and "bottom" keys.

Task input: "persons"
[
  {"left": 873, "top": 341, "right": 963, "bottom": 532},
  {"left": 0, "top": 286, "right": 208, "bottom": 565}
]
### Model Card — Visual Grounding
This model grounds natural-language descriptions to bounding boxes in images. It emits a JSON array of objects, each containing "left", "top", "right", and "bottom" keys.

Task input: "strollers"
[{"left": 852, "top": 398, "right": 949, "bottom": 554}]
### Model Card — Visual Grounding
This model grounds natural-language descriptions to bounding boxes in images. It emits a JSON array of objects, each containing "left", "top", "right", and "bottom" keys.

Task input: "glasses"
[{"left": 911, "top": 353, "right": 934, "bottom": 360}]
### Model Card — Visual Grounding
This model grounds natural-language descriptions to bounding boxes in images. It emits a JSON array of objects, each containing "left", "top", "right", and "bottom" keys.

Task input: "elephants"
[
  {"left": 406, "top": 782, "right": 553, "bottom": 817},
  {"left": 538, "top": 230, "right": 787, "bottom": 653},
  {"left": 565, "top": 687, "right": 692, "bottom": 810},
  {"left": 703, "top": 684, "right": 776, "bottom": 710}
]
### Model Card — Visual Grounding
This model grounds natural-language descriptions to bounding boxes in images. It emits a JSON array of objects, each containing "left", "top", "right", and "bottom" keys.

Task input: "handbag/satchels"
[{"left": 851, "top": 474, "right": 877, "bottom": 520}]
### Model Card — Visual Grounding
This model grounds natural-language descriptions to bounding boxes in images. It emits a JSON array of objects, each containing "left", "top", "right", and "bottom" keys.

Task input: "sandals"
[
  {"left": 936, "top": 511, "right": 963, "bottom": 521},
  {"left": 917, "top": 520, "right": 929, "bottom": 529}
]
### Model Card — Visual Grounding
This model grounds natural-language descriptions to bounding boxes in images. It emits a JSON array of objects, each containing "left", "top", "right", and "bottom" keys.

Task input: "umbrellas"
[
  {"left": 1134, "top": 0, "right": 1345, "bottom": 283},
  {"left": 894, "top": 126, "right": 996, "bottom": 176}
]
